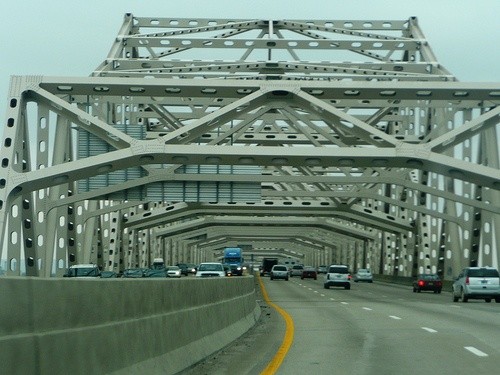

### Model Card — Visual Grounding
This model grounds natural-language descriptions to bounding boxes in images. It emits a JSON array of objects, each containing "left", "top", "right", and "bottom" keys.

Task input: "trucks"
[{"left": 263, "top": 257, "right": 296, "bottom": 275}]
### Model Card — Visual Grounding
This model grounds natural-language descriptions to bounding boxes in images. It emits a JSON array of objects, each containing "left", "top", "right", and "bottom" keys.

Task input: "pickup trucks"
[{"left": 271, "top": 264, "right": 288, "bottom": 282}]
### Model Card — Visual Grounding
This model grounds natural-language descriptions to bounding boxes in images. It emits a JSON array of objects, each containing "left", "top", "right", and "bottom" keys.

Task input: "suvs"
[
  {"left": 452, "top": 267, "right": 500, "bottom": 303},
  {"left": 63, "top": 262, "right": 100, "bottom": 277},
  {"left": 324, "top": 265, "right": 351, "bottom": 290}
]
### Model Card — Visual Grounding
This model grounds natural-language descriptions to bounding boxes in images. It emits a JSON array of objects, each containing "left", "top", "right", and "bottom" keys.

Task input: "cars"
[
  {"left": 354, "top": 268, "right": 373, "bottom": 282},
  {"left": 413, "top": 274, "right": 443, "bottom": 294},
  {"left": 291, "top": 264, "right": 327, "bottom": 280},
  {"left": 100, "top": 258, "right": 242, "bottom": 278}
]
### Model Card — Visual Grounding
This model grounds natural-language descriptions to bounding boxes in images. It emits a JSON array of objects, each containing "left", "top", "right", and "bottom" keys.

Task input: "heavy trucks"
[{"left": 224, "top": 248, "right": 244, "bottom": 275}]
